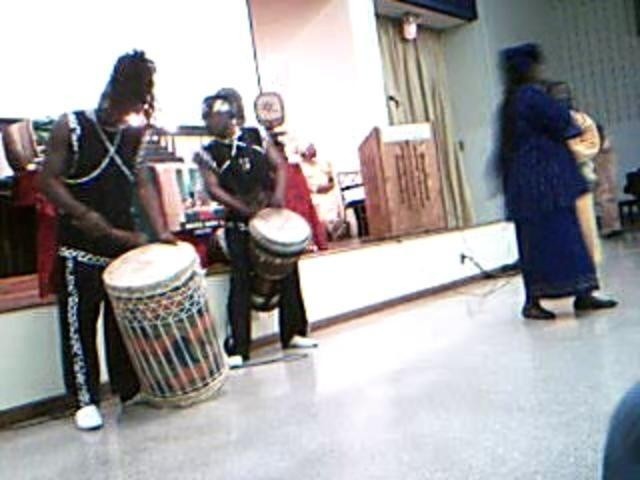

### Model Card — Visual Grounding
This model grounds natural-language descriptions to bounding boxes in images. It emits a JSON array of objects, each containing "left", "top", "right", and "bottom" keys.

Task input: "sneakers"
[
  {"left": 288, "top": 336, "right": 319, "bottom": 349},
  {"left": 572, "top": 296, "right": 618, "bottom": 313},
  {"left": 520, "top": 303, "right": 556, "bottom": 321},
  {"left": 228, "top": 355, "right": 243, "bottom": 368},
  {"left": 73, "top": 404, "right": 102, "bottom": 430}
]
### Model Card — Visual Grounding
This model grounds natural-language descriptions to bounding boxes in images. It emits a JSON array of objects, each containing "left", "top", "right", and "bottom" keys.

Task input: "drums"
[
  {"left": 247, "top": 206, "right": 313, "bottom": 311},
  {"left": 102, "top": 238, "right": 229, "bottom": 408}
]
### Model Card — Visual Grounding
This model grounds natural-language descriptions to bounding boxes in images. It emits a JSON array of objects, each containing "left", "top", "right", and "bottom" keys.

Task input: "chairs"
[
  {"left": 337, "top": 171, "right": 370, "bottom": 237},
  {"left": 619, "top": 170, "right": 639, "bottom": 226}
]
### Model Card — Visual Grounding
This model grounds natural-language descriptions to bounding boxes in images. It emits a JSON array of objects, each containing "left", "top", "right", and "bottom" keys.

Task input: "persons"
[
  {"left": 543, "top": 81, "right": 601, "bottom": 276},
  {"left": 9, "top": 157, "right": 63, "bottom": 298},
  {"left": 37, "top": 49, "right": 181, "bottom": 432},
  {"left": 492, "top": 41, "right": 620, "bottom": 320},
  {"left": 294, "top": 138, "right": 345, "bottom": 245},
  {"left": 191, "top": 94, "right": 321, "bottom": 369},
  {"left": 273, "top": 139, "right": 329, "bottom": 251},
  {"left": 593, "top": 124, "right": 623, "bottom": 240}
]
